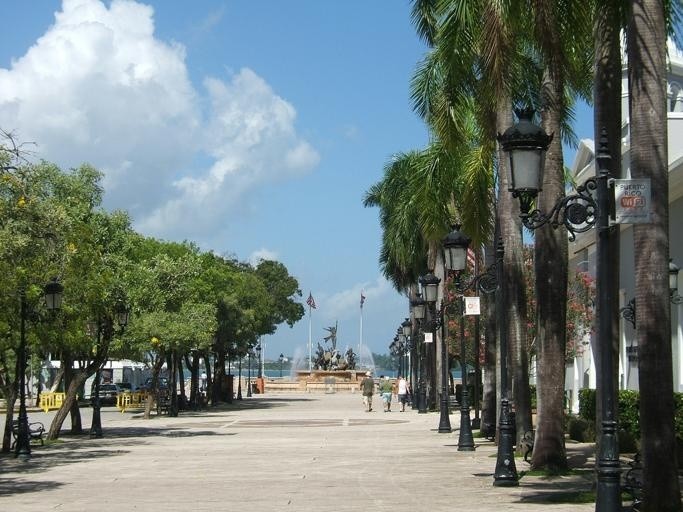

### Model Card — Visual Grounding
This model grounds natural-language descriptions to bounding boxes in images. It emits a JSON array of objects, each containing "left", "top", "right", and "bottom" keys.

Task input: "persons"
[
  {"left": 313, "top": 319, "right": 357, "bottom": 370},
  {"left": 396, "top": 376, "right": 401, "bottom": 402},
  {"left": 379, "top": 375, "right": 384, "bottom": 396},
  {"left": 201, "top": 371, "right": 207, "bottom": 390},
  {"left": 395, "top": 376, "right": 410, "bottom": 412},
  {"left": 360, "top": 371, "right": 374, "bottom": 412},
  {"left": 381, "top": 377, "right": 393, "bottom": 412}
]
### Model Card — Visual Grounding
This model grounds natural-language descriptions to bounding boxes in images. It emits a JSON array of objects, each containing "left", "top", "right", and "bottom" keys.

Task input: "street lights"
[
  {"left": 419, "top": 268, "right": 479, "bottom": 452},
  {"left": 14, "top": 274, "right": 64, "bottom": 462},
  {"left": 497, "top": 105, "right": 626, "bottom": 511},
  {"left": 88, "top": 297, "right": 127, "bottom": 439},
  {"left": 387, "top": 313, "right": 428, "bottom": 415},
  {"left": 442, "top": 204, "right": 522, "bottom": 485},
  {"left": 411, "top": 292, "right": 451, "bottom": 435},
  {"left": 246, "top": 342, "right": 260, "bottom": 398},
  {"left": 279, "top": 352, "right": 283, "bottom": 377}
]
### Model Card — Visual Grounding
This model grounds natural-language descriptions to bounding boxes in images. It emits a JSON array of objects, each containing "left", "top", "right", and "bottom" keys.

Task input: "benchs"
[
  {"left": 6, "top": 416, "right": 45, "bottom": 449},
  {"left": 620, "top": 451, "right": 644, "bottom": 511},
  {"left": 520, "top": 428, "right": 534, "bottom": 461}
]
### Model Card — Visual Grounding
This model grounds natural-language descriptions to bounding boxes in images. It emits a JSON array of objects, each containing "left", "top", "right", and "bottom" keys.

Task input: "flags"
[
  {"left": 306, "top": 295, "right": 316, "bottom": 308},
  {"left": 360, "top": 292, "right": 366, "bottom": 307}
]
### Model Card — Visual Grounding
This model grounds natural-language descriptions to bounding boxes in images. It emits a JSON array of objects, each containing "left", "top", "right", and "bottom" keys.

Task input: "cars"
[
  {"left": 137, "top": 377, "right": 169, "bottom": 399},
  {"left": 113, "top": 382, "right": 140, "bottom": 404},
  {"left": 89, "top": 383, "right": 116, "bottom": 406}
]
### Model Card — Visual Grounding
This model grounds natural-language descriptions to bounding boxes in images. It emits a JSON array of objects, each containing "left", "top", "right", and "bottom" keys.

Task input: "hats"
[{"left": 366, "top": 371, "right": 372, "bottom": 376}]
